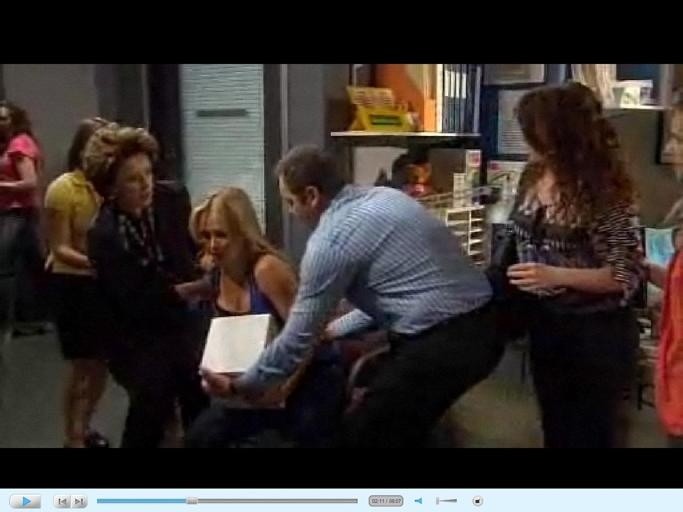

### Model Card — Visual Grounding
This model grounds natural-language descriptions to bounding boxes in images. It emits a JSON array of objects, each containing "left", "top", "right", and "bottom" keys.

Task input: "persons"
[{"left": 0, "top": 80, "right": 683, "bottom": 448}]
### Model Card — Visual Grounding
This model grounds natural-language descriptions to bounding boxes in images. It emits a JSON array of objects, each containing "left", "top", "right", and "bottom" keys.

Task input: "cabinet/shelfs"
[{"left": 441, "top": 204, "right": 484, "bottom": 264}]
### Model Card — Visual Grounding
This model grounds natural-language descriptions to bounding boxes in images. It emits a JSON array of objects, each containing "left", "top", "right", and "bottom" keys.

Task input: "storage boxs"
[{"left": 195, "top": 313, "right": 285, "bottom": 411}]
